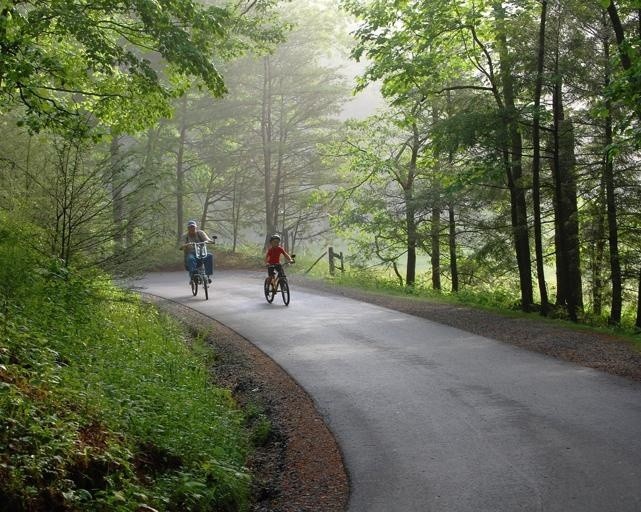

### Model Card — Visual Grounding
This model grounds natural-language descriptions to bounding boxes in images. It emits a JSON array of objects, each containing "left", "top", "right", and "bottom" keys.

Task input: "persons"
[
  {"left": 264, "top": 235, "right": 295, "bottom": 295},
  {"left": 180, "top": 220, "right": 214, "bottom": 285}
]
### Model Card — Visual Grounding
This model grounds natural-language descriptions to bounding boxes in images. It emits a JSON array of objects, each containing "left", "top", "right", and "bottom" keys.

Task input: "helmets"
[{"left": 271, "top": 235, "right": 281, "bottom": 242}]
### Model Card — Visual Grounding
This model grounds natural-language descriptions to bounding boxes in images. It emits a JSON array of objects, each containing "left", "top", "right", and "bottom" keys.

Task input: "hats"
[{"left": 188, "top": 221, "right": 197, "bottom": 228}]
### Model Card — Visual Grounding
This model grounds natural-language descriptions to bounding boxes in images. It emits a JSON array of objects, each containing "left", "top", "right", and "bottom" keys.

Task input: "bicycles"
[
  {"left": 265, "top": 255, "right": 296, "bottom": 306},
  {"left": 185, "top": 235, "right": 217, "bottom": 299}
]
